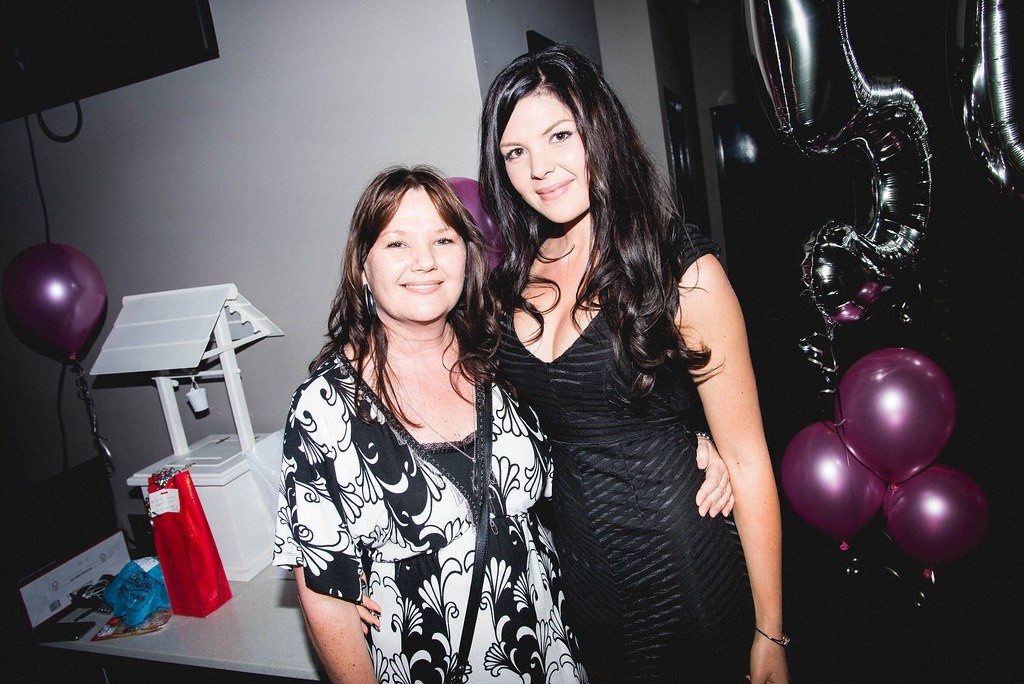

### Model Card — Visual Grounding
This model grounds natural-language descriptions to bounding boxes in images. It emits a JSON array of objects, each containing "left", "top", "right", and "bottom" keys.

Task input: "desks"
[{"left": 37, "top": 561, "right": 329, "bottom": 684}]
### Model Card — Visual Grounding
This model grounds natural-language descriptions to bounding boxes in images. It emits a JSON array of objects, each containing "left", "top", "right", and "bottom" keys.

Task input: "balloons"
[
  {"left": 436, "top": 174, "right": 509, "bottom": 272},
  {"left": 0, "top": 244, "right": 106, "bottom": 361}
]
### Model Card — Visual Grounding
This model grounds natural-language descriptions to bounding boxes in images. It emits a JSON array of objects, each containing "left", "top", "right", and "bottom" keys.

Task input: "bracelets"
[
  {"left": 695, "top": 430, "right": 713, "bottom": 442},
  {"left": 755, "top": 627, "right": 789, "bottom": 647}
]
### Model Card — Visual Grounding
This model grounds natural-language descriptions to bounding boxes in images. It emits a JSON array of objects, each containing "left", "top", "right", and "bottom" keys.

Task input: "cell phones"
[{"left": 33, "top": 621, "right": 95, "bottom": 643}]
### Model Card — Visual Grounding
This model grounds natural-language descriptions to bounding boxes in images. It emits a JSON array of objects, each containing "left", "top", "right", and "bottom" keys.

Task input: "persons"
[
  {"left": 270, "top": 166, "right": 735, "bottom": 684},
  {"left": 354, "top": 45, "right": 789, "bottom": 684}
]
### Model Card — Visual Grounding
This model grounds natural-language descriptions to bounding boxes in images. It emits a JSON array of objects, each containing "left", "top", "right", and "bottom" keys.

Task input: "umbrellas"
[{"left": 780, "top": 348, "right": 988, "bottom": 578}]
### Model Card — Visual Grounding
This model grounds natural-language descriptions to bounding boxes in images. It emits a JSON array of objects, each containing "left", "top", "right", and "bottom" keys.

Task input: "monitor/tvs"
[{"left": 0, "top": 0, "right": 220, "bottom": 124}]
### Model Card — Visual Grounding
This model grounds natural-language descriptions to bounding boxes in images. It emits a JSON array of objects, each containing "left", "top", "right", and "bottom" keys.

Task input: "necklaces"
[{"left": 390, "top": 371, "right": 477, "bottom": 463}]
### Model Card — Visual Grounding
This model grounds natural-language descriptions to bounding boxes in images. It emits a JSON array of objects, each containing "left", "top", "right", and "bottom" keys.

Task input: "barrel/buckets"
[{"left": 186, "top": 380, "right": 209, "bottom": 413}]
